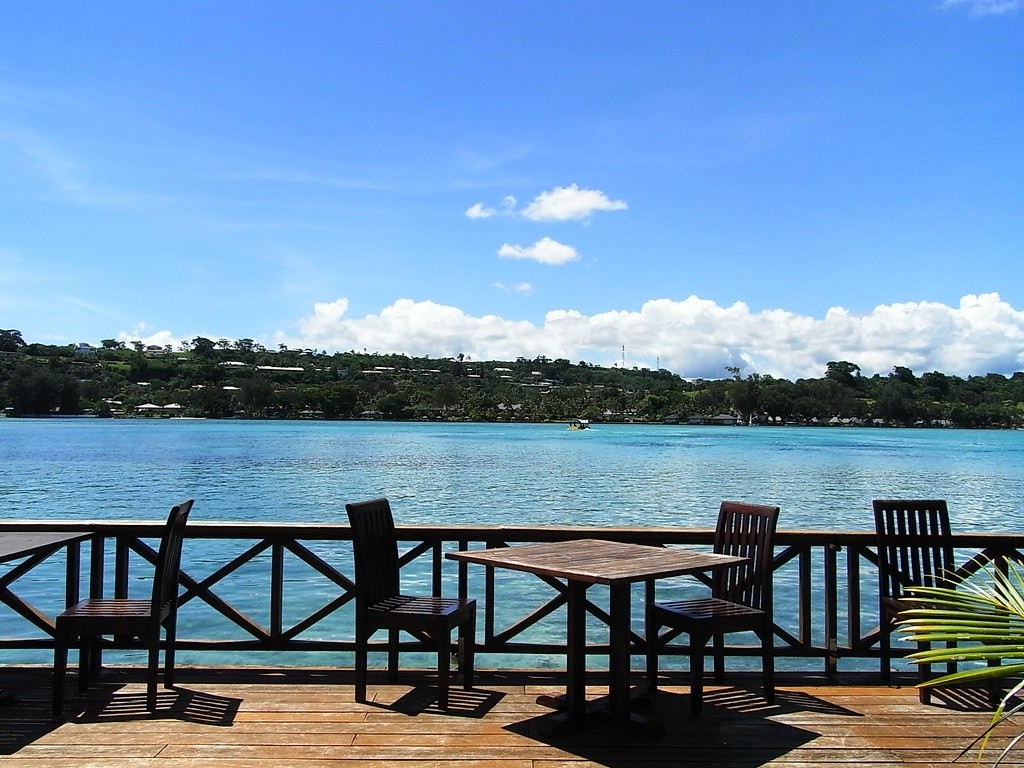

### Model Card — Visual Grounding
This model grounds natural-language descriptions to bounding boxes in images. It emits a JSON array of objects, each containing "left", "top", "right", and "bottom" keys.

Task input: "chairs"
[
  {"left": 643, "top": 501, "right": 781, "bottom": 713},
  {"left": 872, "top": 498, "right": 1006, "bottom": 707},
  {"left": 347, "top": 496, "right": 478, "bottom": 710},
  {"left": 53, "top": 499, "right": 194, "bottom": 716}
]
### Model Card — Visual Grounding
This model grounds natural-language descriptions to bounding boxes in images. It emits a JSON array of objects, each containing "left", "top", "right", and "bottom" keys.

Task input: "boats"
[{"left": 567, "top": 419, "right": 590, "bottom": 431}]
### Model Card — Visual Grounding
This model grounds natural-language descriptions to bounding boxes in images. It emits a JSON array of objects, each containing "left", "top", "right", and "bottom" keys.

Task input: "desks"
[
  {"left": 0, "top": 531, "right": 96, "bottom": 563},
  {"left": 445, "top": 538, "right": 751, "bottom": 739}
]
